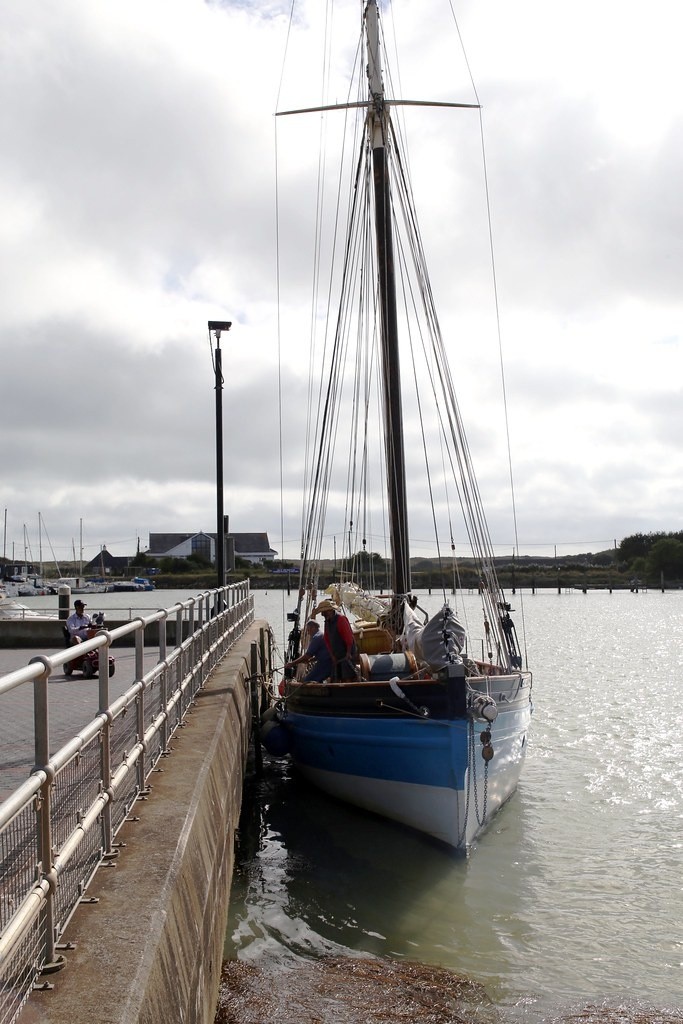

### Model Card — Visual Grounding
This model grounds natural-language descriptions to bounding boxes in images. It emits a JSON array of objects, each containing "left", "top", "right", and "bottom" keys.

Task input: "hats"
[
  {"left": 74, "top": 600, "right": 86, "bottom": 606},
  {"left": 314, "top": 601, "right": 341, "bottom": 615}
]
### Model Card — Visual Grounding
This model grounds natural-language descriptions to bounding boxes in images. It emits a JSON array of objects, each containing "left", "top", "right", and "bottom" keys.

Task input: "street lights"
[{"left": 205, "top": 320, "right": 233, "bottom": 619}]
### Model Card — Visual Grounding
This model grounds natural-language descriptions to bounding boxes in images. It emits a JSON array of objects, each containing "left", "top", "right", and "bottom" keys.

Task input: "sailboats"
[
  {"left": 251, "top": 0, "right": 539, "bottom": 861},
  {"left": 0, "top": 507, "right": 157, "bottom": 621}
]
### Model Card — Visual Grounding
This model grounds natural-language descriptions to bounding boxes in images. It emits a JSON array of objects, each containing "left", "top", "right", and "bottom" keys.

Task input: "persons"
[
  {"left": 315, "top": 601, "right": 358, "bottom": 683},
  {"left": 284, "top": 619, "right": 331, "bottom": 683},
  {"left": 66, "top": 600, "right": 92, "bottom": 647}
]
